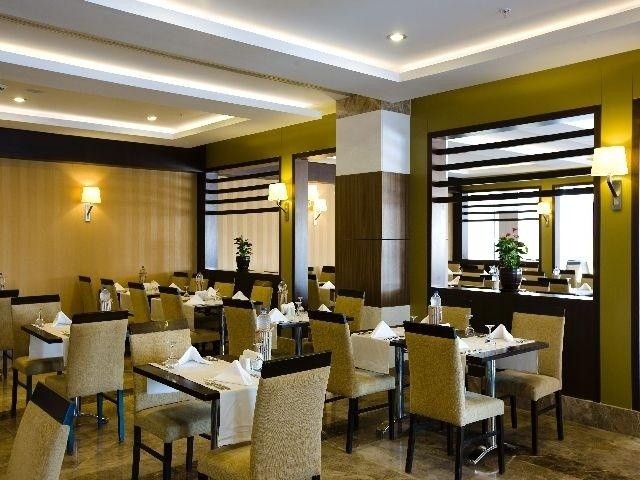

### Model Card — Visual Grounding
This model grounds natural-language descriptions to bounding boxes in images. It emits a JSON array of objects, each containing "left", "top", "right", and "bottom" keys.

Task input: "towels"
[
  {"left": 318, "top": 303, "right": 331, "bottom": 312},
  {"left": 179, "top": 345, "right": 206, "bottom": 366},
  {"left": 268, "top": 308, "right": 288, "bottom": 322},
  {"left": 371, "top": 321, "right": 398, "bottom": 339},
  {"left": 114, "top": 283, "right": 125, "bottom": 291},
  {"left": 490, "top": 324, "right": 513, "bottom": 342},
  {"left": 232, "top": 291, "right": 249, "bottom": 301},
  {"left": 51, "top": 311, "right": 72, "bottom": 326},
  {"left": 575, "top": 283, "right": 592, "bottom": 294},
  {"left": 213, "top": 359, "right": 251, "bottom": 386},
  {"left": 184, "top": 295, "right": 203, "bottom": 304},
  {"left": 169, "top": 283, "right": 181, "bottom": 292},
  {"left": 322, "top": 281, "right": 335, "bottom": 288}
]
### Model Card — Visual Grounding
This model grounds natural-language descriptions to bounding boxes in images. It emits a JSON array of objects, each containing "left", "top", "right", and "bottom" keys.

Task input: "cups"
[
  {"left": 183, "top": 285, "right": 189, "bottom": 298},
  {"left": 464, "top": 313, "right": 475, "bottom": 335},
  {"left": 35, "top": 310, "right": 45, "bottom": 328},
  {"left": 253, "top": 342, "right": 264, "bottom": 371},
  {"left": 166, "top": 345, "right": 178, "bottom": 369},
  {"left": 297, "top": 296, "right": 304, "bottom": 306},
  {"left": 295, "top": 302, "right": 302, "bottom": 322},
  {"left": 410, "top": 315, "right": 418, "bottom": 322},
  {"left": 566, "top": 277, "right": 572, "bottom": 287},
  {"left": 484, "top": 324, "right": 496, "bottom": 348}
]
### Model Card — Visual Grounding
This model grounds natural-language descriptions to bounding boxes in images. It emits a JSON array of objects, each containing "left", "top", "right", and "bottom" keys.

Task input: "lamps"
[
  {"left": 538, "top": 201, "right": 551, "bottom": 227},
  {"left": 268, "top": 182, "right": 289, "bottom": 222},
  {"left": 81, "top": 186, "right": 101, "bottom": 221},
  {"left": 313, "top": 199, "right": 326, "bottom": 225},
  {"left": 590, "top": 147, "right": 627, "bottom": 211},
  {"left": 308, "top": 182, "right": 317, "bottom": 211}
]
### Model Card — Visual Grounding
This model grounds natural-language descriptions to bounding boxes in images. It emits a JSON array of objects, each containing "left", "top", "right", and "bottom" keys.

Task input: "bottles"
[
  {"left": 195, "top": 272, "right": 203, "bottom": 293},
  {"left": 138, "top": 267, "right": 146, "bottom": 284},
  {"left": 257, "top": 308, "right": 272, "bottom": 361},
  {"left": 491, "top": 272, "right": 499, "bottom": 292},
  {"left": 0, "top": 273, "right": 5, "bottom": 291},
  {"left": 432, "top": 292, "right": 442, "bottom": 324},
  {"left": 100, "top": 287, "right": 110, "bottom": 312},
  {"left": 277, "top": 279, "right": 288, "bottom": 311}
]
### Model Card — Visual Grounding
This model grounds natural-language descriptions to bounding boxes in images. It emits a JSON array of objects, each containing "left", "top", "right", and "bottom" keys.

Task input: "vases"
[
  {"left": 498, "top": 269, "right": 521, "bottom": 293},
  {"left": 236, "top": 257, "right": 248, "bottom": 271}
]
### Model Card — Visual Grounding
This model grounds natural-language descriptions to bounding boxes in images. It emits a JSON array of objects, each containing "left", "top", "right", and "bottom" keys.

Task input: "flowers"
[
  {"left": 233, "top": 234, "right": 254, "bottom": 257},
  {"left": 495, "top": 227, "right": 528, "bottom": 268}
]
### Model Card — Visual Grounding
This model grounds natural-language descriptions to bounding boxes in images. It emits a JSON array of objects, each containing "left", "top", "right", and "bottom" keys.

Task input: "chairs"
[
  {"left": 222, "top": 297, "right": 265, "bottom": 357},
  {"left": 0, "top": 288, "right": 19, "bottom": 378},
  {"left": 402, "top": 319, "right": 505, "bottom": 480},
  {"left": 335, "top": 288, "right": 365, "bottom": 331},
  {"left": 172, "top": 271, "right": 189, "bottom": 291},
  {"left": 189, "top": 272, "right": 211, "bottom": 294},
  {"left": 319, "top": 266, "right": 335, "bottom": 285},
  {"left": 157, "top": 286, "right": 220, "bottom": 356},
  {"left": 308, "top": 266, "right": 316, "bottom": 277},
  {"left": 78, "top": 275, "right": 99, "bottom": 312},
  {"left": 198, "top": 350, "right": 333, "bottom": 479},
  {"left": 308, "top": 274, "right": 332, "bottom": 310},
  {"left": 308, "top": 310, "right": 397, "bottom": 452},
  {"left": 251, "top": 279, "right": 279, "bottom": 313},
  {"left": 214, "top": 270, "right": 236, "bottom": 299},
  {"left": 426, "top": 305, "right": 471, "bottom": 332},
  {"left": 128, "top": 281, "right": 162, "bottom": 332},
  {"left": 11, "top": 295, "right": 61, "bottom": 414},
  {"left": 100, "top": 278, "right": 133, "bottom": 330},
  {"left": 46, "top": 311, "right": 133, "bottom": 452},
  {"left": 481, "top": 306, "right": 565, "bottom": 454},
  {"left": 126, "top": 316, "right": 211, "bottom": 480},
  {"left": 2, "top": 380, "right": 79, "bottom": 479}
]
